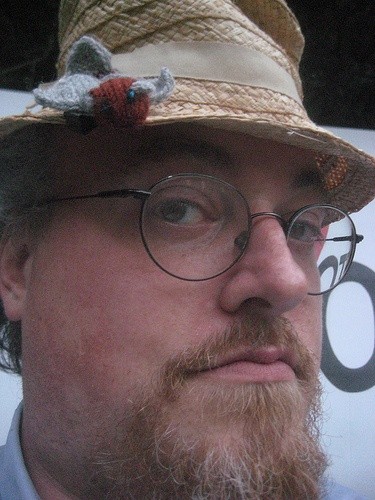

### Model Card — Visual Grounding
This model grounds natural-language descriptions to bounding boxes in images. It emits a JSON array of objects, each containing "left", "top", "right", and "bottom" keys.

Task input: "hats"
[{"left": 0, "top": 0, "right": 374, "bottom": 218}]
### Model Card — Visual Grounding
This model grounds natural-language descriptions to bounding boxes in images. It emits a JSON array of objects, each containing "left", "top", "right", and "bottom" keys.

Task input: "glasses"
[{"left": 29, "top": 171, "right": 363, "bottom": 296}]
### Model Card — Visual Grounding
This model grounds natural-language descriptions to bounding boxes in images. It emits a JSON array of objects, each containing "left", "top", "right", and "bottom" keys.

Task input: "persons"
[{"left": 0, "top": 0, "right": 375, "bottom": 500}]
